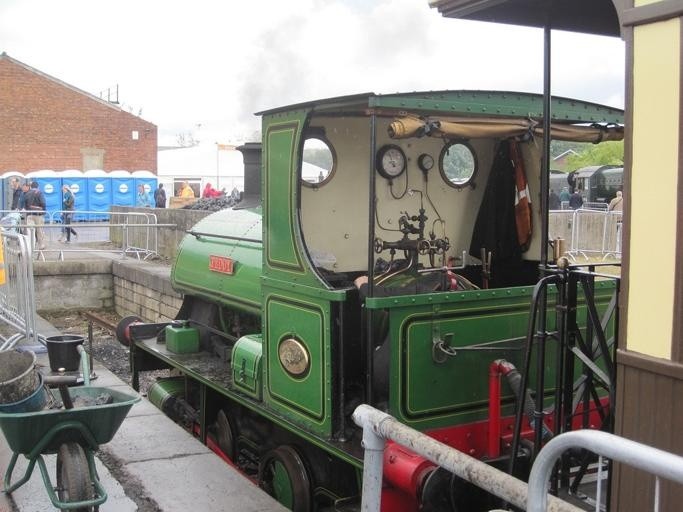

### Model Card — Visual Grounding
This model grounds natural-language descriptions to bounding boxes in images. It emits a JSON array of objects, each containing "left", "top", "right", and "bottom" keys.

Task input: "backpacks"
[{"left": 157, "top": 189, "right": 165, "bottom": 207}]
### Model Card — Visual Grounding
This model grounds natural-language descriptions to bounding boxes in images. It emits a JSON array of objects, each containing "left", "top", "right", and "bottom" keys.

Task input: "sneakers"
[{"left": 64, "top": 232, "right": 80, "bottom": 243}]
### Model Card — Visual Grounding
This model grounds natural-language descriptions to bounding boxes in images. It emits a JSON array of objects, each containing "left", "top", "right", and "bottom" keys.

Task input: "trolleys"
[{"left": 0, "top": 344, "right": 141, "bottom": 512}]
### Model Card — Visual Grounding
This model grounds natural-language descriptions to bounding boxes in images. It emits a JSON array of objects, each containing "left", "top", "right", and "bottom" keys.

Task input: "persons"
[
  {"left": 156, "top": 189, "right": 166, "bottom": 208},
  {"left": 608, "top": 191, "right": 623, "bottom": 212},
  {"left": 10, "top": 177, "right": 47, "bottom": 250},
  {"left": 179, "top": 182, "right": 194, "bottom": 198},
  {"left": 548, "top": 185, "right": 583, "bottom": 212},
  {"left": 203, "top": 183, "right": 227, "bottom": 198},
  {"left": 61, "top": 184, "right": 79, "bottom": 244},
  {"left": 154, "top": 184, "right": 165, "bottom": 207}
]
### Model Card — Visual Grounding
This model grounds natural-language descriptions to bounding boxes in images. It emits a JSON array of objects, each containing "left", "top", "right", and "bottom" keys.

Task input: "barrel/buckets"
[
  {"left": 1, "top": 349, "right": 41, "bottom": 402},
  {"left": 1, "top": 369, "right": 50, "bottom": 412},
  {"left": 46, "top": 335, "right": 84, "bottom": 371}
]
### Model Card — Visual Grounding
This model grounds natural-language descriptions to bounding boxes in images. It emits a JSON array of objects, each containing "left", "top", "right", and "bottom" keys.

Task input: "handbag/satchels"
[{"left": 30, "top": 205, "right": 43, "bottom": 214}]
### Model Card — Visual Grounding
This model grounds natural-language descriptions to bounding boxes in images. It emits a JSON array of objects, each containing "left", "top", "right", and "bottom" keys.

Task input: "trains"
[
  {"left": 112, "top": 0, "right": 681, "bottom": 512},
  {"left": 548, "top": 164, "right": 623, "bottom": 209}
]
